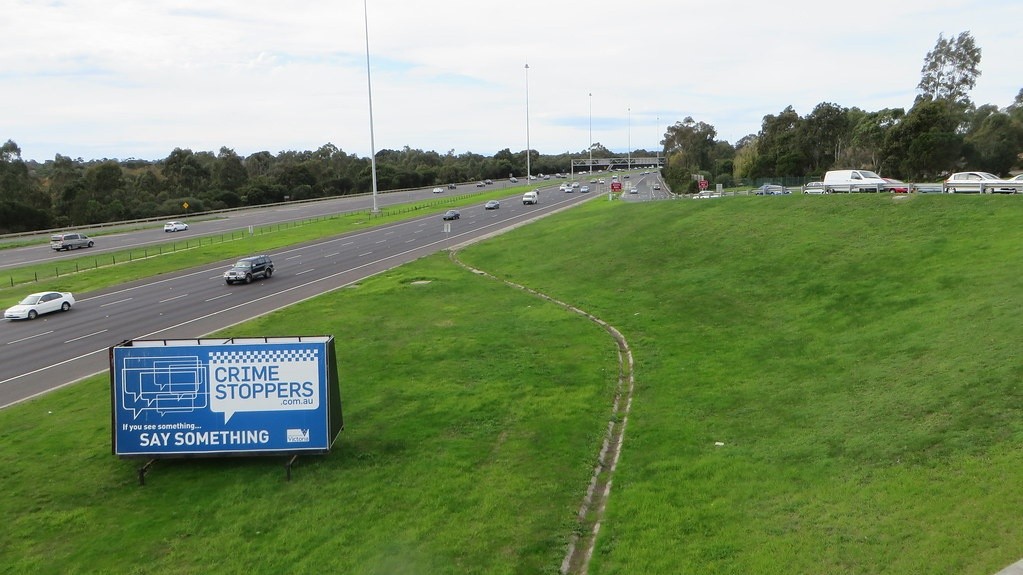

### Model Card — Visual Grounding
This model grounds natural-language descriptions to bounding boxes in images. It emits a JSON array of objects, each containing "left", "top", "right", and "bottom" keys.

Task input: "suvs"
[
  {"left": 223, "top": 254, "right": 275, "bottom": 284},
  {"left": 944, "top": 171, "right": 1016, "bottom": 195}
]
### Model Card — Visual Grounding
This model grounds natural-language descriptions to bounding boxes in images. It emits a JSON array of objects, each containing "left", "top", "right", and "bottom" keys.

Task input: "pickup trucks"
[{"left": 692, "top": 190, "right": 721, "bottom": 199}]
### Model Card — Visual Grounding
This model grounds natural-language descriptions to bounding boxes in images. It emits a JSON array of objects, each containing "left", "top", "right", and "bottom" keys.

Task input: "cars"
[
  {"left": 485, "top": 200, "right": 500, "bottom": 210},
  {"left": 510, "top": 177, "right": 518, "bottom": 183},
  {"left": 443, "top": 210, "right": 460, "bottom": 220},
  {"left": 754, "top": 185, "right": 791, "bottom": 195},
  {"left": 50, "top": 233, "right": 95, "bottom": 251},
  {"left": 881, "top": 178, "right": 913, "bottom": 193},
  {"left": 526, "top": 164, "right": 659, "bottom": 194},
  {"left": 629, "top": 187, "right": 638, "bottom": 194},
  {"left": 433, "top": 187, "right": 444, "bottom": 193},
  {"left": 531, "top": 189, "right": 540, "bottom": 195},
  {"left": 485, "top": 179, "right": 493, "bottom": 185},
  {"left": 1007, "top": 174, "right": 1023, "bottom": 194},
  {"left": 476, "top": 182, "right": 486, "bottom": 187},
  {"left": 4, "top": 292, "right": 75, "bottom": 320},
  {"left": 580, "top": 185, "right": 590, "bottom": 192},
  {"left": 164, "top": 220, "right": 189, "bottom": 232},
  {"left": 653, "top": 183, "right": 660, "bottom": 190},
  {"left": 564, "top": 187, "right": 575, "bottom": 193},
  {"left": 447, "top": 184, "right": 457, "bottom": 190}
]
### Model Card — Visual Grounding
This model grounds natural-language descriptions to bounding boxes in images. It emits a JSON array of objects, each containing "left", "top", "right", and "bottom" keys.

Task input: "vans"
[
  {"left": 803, "top": 181, "right": 827, "bottom": 194},
  {"left": 823, "top": 170, "right": 888, "bottom": 193}
]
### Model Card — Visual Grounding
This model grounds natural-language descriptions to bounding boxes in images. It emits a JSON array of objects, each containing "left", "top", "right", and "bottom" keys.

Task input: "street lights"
[
  {"left": 656, "top": 117, "right": 660, "bottom": 167},
  {"left": 628, "top": 107, "right": 631, "bottom": 172},
  {"left": 524, "top": 63, "right": 531, "bottom": 185},
  {"left": 588, "top": 92, "right": 593, "bottom": 175}
]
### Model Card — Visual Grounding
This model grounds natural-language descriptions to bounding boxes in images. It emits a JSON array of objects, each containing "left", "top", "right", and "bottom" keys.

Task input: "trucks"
[{"left": 522, "top": 191, "right": 538, "bottom": 205}]
